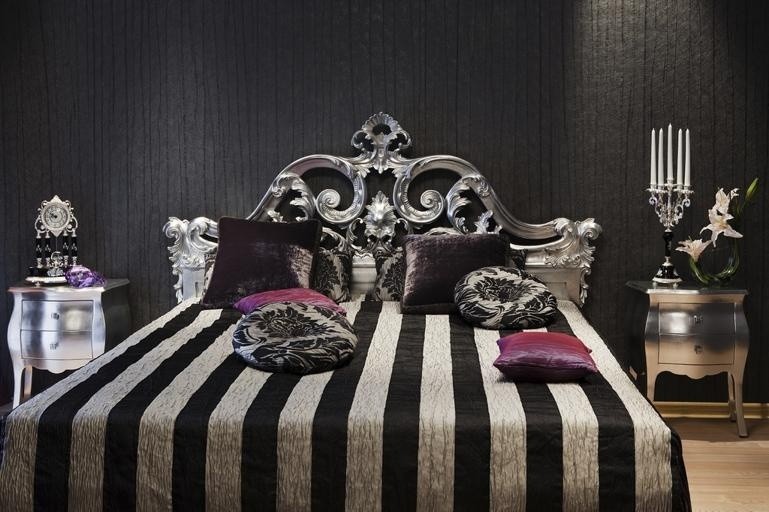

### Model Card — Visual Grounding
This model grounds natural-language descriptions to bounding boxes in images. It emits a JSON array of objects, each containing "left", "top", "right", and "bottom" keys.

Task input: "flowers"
[{"left": 674, "top": 176, "right": 760, "bottom": 264}]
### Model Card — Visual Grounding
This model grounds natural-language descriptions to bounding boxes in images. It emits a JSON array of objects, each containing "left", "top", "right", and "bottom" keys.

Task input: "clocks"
[{"left": 34, "top": 195, "right": 78, "bottom": 277}]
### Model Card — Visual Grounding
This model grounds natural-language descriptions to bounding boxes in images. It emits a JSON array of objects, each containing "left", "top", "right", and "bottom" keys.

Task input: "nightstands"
[
  {"left": 627, "top": 280, "right": 751, "bottom": 437},
  {"left": 6, "top": 279, "right": 132, "bottom": 410}
]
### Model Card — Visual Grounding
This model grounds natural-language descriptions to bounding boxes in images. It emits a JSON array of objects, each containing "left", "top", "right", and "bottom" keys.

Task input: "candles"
[{"left": 650, "top": 122, "right": 690, "bottom": 187}]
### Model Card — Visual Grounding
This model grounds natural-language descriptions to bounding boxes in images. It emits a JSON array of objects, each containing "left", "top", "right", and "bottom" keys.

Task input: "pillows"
[
  {"left": 401, "top": 234, "right": 510, "bottom": 313},
  {"left": 493, "top": 332, "right": 597, "bottom": 378},
  {"left": 204, "top": 246, "right": 353, "bottom": 302},
  {"left": 371, "top": 249, "right": 528, "bottom": 302},
  {"left": 454, "top": 266, "right": 557, "bottom": 330},
  {"left": 203, "top": 215, "right": 323, "bottom": 309},
  {"left": 234, "top": 287, "right": 348, "bottom": 318},
  {"left": 232, "top": 301, "right": 359, "bottom": 373}
]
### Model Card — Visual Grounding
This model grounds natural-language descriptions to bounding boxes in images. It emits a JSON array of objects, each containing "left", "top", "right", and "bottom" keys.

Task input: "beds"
[{"left": 0, "top": 112, "right": 693, "bottom": 511}]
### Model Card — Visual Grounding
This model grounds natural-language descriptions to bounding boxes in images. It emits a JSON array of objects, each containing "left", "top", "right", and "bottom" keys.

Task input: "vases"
[{"left": 690, "top": 245, "right": 740, "bottom": 285}]
